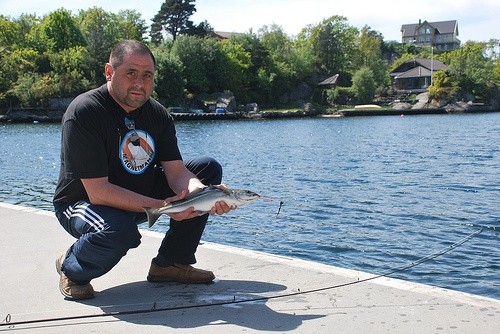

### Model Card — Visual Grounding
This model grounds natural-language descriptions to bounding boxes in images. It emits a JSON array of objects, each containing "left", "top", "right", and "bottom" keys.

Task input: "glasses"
[{"left": 124, "top": 114, "right": 140, "bottom": 147}]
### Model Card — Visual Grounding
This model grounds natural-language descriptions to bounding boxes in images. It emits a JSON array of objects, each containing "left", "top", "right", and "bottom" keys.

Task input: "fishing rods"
[{"left": 0, "top": 228, "right": 483, "bottom": 327}]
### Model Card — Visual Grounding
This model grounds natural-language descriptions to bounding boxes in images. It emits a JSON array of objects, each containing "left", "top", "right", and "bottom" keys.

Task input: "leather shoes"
[
  {"left": 147, "top": 257, "right": 216, "bottom": 285},
  {"left": 56, "top": 255, "right": 95, "bottom": 298}
]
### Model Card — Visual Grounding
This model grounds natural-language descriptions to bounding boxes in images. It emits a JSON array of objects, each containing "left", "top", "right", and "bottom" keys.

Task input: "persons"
[{"left": 52, "top": 39, "right": 237, "bottom": 298}]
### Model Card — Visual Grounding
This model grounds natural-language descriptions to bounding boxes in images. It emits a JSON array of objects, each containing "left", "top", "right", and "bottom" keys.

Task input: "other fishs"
[{"left": 141, "top": 184, "right": 260, "bottom": 229}]
